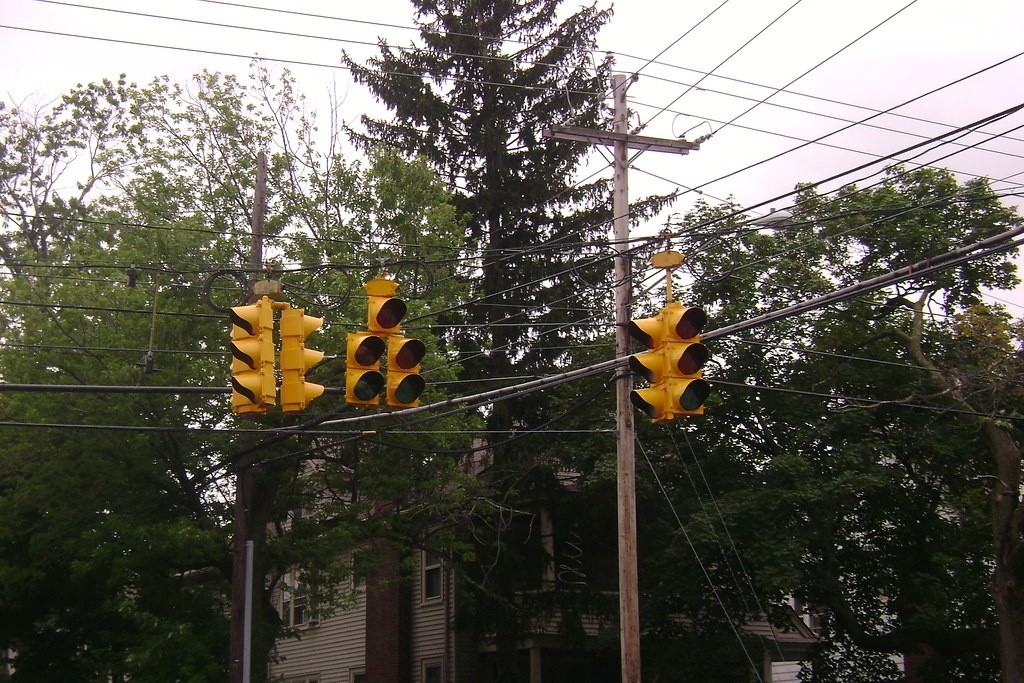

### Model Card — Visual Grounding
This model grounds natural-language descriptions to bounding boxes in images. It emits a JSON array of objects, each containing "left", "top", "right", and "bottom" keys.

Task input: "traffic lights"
[
  {"left": 626, "top": 309, "right": 674, "bottom": 423},
  {"left": 344, "top": 332, "right": 385, "bottom": 406},
  {"left": 278, "top": 309, "right": 326, "bottom": 414},
  {"left": 226, "top": 301, "right": 278, "bottom": 416},
  {"left": 664, "top": 300, "right": 711, "bottom": 414},
  {"left": 367, "top": 295, "right": 408, "bottom": 334},
  {"left": 385, "top": 335, "right": 427, "bottom": 409}
]
